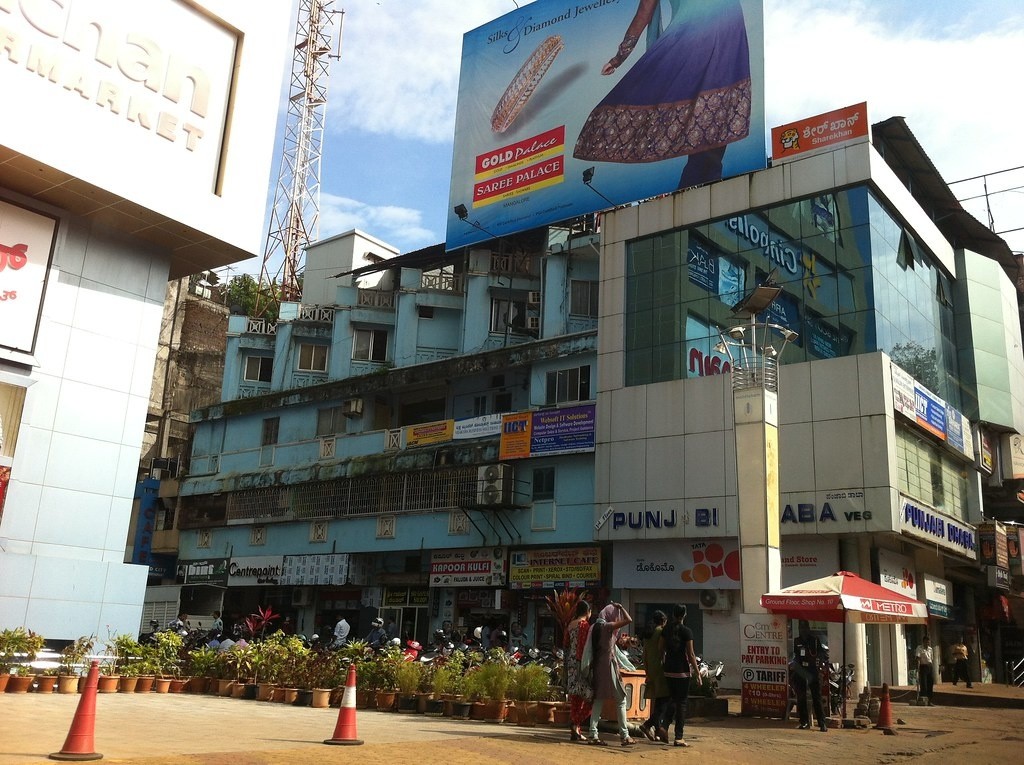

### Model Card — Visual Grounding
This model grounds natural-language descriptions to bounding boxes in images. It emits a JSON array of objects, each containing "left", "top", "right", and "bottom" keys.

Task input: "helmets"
[{"left": 372, "top": 618, "right": 384, "bottom": 627}]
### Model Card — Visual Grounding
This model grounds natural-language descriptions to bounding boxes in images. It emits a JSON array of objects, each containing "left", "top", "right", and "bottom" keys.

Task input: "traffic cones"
[
  {"left": 871, "top": 682, "right": 898, "bottom": 731},
  {"left": 322, "top": 663, "right": 364, "bottom": 746},
  {"left": 47, "top": 659, "right": 105, "bottom": 761}
]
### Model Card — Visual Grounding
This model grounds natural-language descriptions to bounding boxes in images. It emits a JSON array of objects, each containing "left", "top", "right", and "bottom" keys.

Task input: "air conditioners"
[
  {"left": 529, "top": 291, "right": 540, "bottom": 303},
  {"left": 476, "top": 463, "right": 515, "bottom": 503},
  {"left": 528, "top": 317, "right": 539, "bottom": 329}
]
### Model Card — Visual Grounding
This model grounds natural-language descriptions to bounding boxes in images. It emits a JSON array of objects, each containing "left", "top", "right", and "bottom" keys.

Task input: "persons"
[
  {"left": 915, "top": 636, "right": 935, "bottom": 707},
  {"left": 169, "top": 611, "right": 191, "bottom": 634},
  {"left": 209, "top": 610, "right": 224, "bottom": 635},
  {"left": 789, "top": 619, "right": 829, "bottom": 732},
  {"left": 296, "top": 611, "right": 397, "bottom": 651},
  {"left": 569, "top": 0, "right": 752, "bottom": 195},
  {"left": 946, "top": 633, "right": 974, "bottom": 690},
  {"left": 204, "top": 618, "right": 249, "bottom": 651},
  {"left": 566, "top": 600, "right": 703, "bottom": 748},
  {"left": 481, "top": 619, "right": 507, "bottom": 652}
]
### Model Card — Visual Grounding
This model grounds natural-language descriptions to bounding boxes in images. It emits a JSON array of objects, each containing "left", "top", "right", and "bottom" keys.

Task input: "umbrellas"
[{"left": 758, "top": 569, "right": 930, "bottom": 721}]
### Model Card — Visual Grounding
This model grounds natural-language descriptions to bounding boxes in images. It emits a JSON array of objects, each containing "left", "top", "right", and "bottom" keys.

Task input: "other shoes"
[
  {"left": 953, "top": 682, "right": 956, "bottom": 685},
  {"left": 658, "top": 727, "right": 669, "bottom": 743},
  {"left": 799, "top": 723, "right": 810, "bottom": 729},
  {"left": 674, "top": 741, "right": 690, "bottom": 747},
  {"left": 928, "top": 702, "right": 933, "bottom": 706},
  {"left": 571, "top": 725, "right": 589, "bottom": 742},
  {"left": 966, "top": 685, "right": 973, "bottom": 688},
  {"left": 639, "top": 723, "right": 654, "bottom": 740},
  {"left": 820, "top": 726, "right": 827, "bottom": 732}
]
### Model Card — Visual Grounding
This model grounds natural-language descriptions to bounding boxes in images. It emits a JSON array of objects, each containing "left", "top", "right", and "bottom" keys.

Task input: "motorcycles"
[{"left": 137, "top": 617, "right": 855, "bottom": 714}]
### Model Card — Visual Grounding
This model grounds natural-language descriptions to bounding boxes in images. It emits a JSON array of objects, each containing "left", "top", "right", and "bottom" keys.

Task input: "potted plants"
[{"left": 0, "top": 627, "right": 573, "bottom": 728}]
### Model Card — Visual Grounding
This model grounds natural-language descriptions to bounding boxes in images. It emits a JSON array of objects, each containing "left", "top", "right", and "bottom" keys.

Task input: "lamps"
[
  {"left": 582, "top": 166, "right": 619, "bottom": 208},
  {"left": 350, "top": 392, "right": 363, "bottom": 414},
  {"left": 453, "top": 204, "right": 514, "bottom": 247}
]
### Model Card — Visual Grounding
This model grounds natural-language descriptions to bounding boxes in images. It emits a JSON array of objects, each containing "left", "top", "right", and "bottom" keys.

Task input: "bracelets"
[{"left": 490, "top": 34, "right": 566, "bottom": 133}]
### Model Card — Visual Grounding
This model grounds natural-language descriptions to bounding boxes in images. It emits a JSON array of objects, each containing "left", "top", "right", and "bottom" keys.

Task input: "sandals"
[
  {"left": 588, "top": 739, "right": 607, "bottom": 746},
  {"left": 621, "top": 738, "right": 638, "bottom": 745}
]
